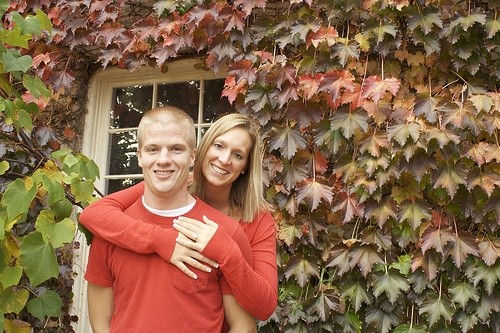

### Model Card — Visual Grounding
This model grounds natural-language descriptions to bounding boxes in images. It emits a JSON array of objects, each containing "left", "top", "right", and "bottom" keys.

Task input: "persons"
[
  {"left": 78, "top": 113, "right": 279, "bottom": 332},
  {"left": 84, "top": 105, "right": 256, "bottom": 332}
]
